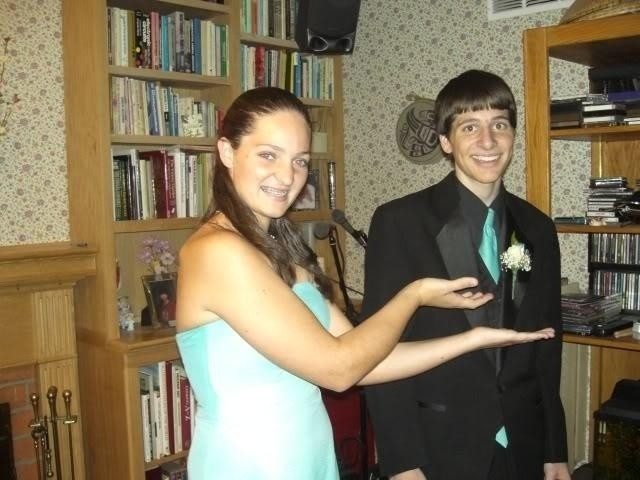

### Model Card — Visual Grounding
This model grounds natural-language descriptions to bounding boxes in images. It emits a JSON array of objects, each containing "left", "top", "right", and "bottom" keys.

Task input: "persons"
[
  {"left": 362, "top": 70, "right": 572, "bottom": 480},
  {"left": 173, "top": 86, "right": 556, "bottom": 479}
]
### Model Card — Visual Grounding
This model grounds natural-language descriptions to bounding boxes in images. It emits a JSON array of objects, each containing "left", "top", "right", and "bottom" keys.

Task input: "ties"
[{"left": 479, "top": 207, "right": 501, "bottom": 285}]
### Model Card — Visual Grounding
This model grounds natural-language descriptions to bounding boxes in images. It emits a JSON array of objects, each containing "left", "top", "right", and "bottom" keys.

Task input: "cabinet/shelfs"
[
  {"left": 522, "top": 11, "right": 640, "bottom": 465},
  {"left": 60, "top": 0, "right": 363, "bottom": 480}
]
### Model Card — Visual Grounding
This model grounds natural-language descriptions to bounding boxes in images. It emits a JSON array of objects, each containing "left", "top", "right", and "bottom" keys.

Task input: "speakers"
[{"left": 295, "top": 0, "right": 361, "bottom": 55}]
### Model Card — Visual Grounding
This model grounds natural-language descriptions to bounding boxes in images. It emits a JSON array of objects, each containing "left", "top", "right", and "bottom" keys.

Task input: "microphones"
[
  {"left": 331, "top": 209, "right": 366, "bottom": 249},
  {"left": 314, "top": 222, "right": 336, "bottom": 239}
]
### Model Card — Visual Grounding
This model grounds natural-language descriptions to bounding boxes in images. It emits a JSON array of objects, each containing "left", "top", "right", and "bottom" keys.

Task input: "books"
[{"left": 549, "top": 61, "right": 639, "bottom": 343}]
